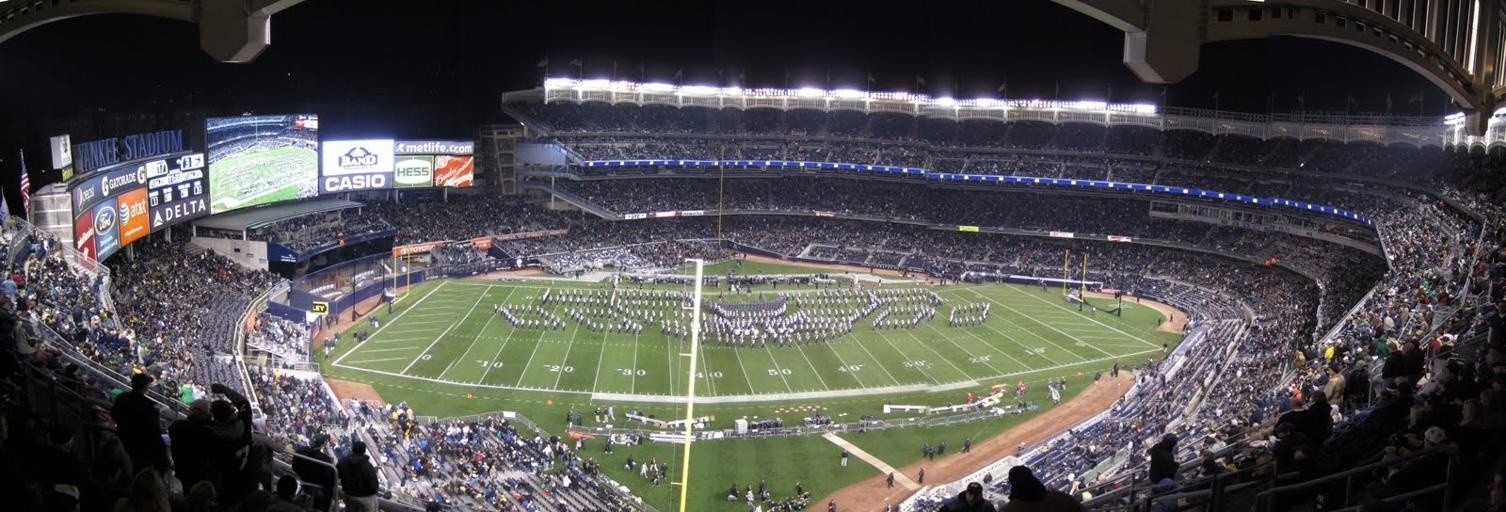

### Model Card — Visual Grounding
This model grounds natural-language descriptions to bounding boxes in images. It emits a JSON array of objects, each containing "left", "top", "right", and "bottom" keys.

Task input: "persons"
[{"left": 0, "top": 107, "right": 1505, "bottom": 512}]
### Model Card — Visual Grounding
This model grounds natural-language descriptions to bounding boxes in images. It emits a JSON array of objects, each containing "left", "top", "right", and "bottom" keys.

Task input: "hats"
[
  {"left": 189, "top": 398, "right": 208, "bottom": 415},
  {"left": 309, "top": 434, "right": 325, "bottom": 448},
  {"left": 211, "top": 400, "right": 229, "bottom": 416},
  {"left": 132, "top": 373, "right": 154, "bottom": 387}
]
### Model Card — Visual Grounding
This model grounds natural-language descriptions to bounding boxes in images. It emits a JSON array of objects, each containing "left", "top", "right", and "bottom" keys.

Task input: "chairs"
[
  {"left": 896, "top": 192, "right": 1506, "bottom": 512},
  {"left": 0, "top": 213, "right": 656, "bottom": 512}
]
